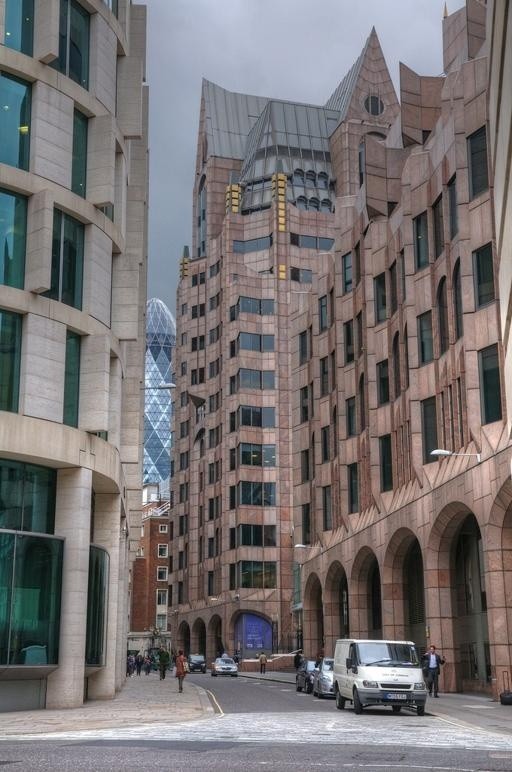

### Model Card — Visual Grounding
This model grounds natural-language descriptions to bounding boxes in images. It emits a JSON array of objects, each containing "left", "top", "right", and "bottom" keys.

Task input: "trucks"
[{"left": 333, "top": 638, "right": 427, "bottom": 716}]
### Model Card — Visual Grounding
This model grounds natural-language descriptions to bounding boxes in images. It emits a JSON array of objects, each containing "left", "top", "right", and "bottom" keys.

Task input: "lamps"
[
  {"left": 211, "top": 598, "right": 226, "bottom": 602},
  {"left": 294, "top": 544, "right": 323, "bottom": 553},
  {"left": 430, "top": 449, "right": 481, "bottom": 464},
  {"left": 141, "top": 383, "right": 177, "bottom": 390}
]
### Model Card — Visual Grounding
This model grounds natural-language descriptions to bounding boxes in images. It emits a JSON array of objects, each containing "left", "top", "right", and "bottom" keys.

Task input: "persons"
[
  {"left": 259, "top": 651, "right": 267, "bottom": 674},
  {"left": 293, "top": 652, "right": 302, "bottom": 672},
  {"left": 206, "top": 648, "right": 240, "bottom": 673},
  {"left": 421, "top": 645, "right": 446, "bottom": 698},
  {"left": 127, "top": 646, "right": 177, "bottom": 681},
  {"left": 175, "top": 649, "right": 186, "bottom": 693}
]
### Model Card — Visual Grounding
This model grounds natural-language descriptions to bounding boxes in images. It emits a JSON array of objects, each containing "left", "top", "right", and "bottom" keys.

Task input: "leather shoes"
[{"left": 434, "top": 696, "right": 439, "bottom": 698}]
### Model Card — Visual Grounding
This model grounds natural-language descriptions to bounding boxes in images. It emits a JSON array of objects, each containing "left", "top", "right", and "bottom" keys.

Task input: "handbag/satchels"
[{"left": 183, "top": 662, "right": 190, "bottom": 673}]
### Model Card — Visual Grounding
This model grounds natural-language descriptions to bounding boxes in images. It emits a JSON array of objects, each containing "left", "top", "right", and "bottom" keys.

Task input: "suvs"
[{"left": 187, "top": 654, "right": 207, "bottom": 673}]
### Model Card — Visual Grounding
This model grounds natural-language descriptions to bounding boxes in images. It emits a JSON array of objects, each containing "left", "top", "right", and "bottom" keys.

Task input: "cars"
[
  {"left": 295, "top": 658, "right": 335, "bottom": 698},
  {"left": 153, "top": 655, "right": 173, "bottom": 671},
  {"left": 211, "top": 657, "right": 238, "bottom": 677}
]
